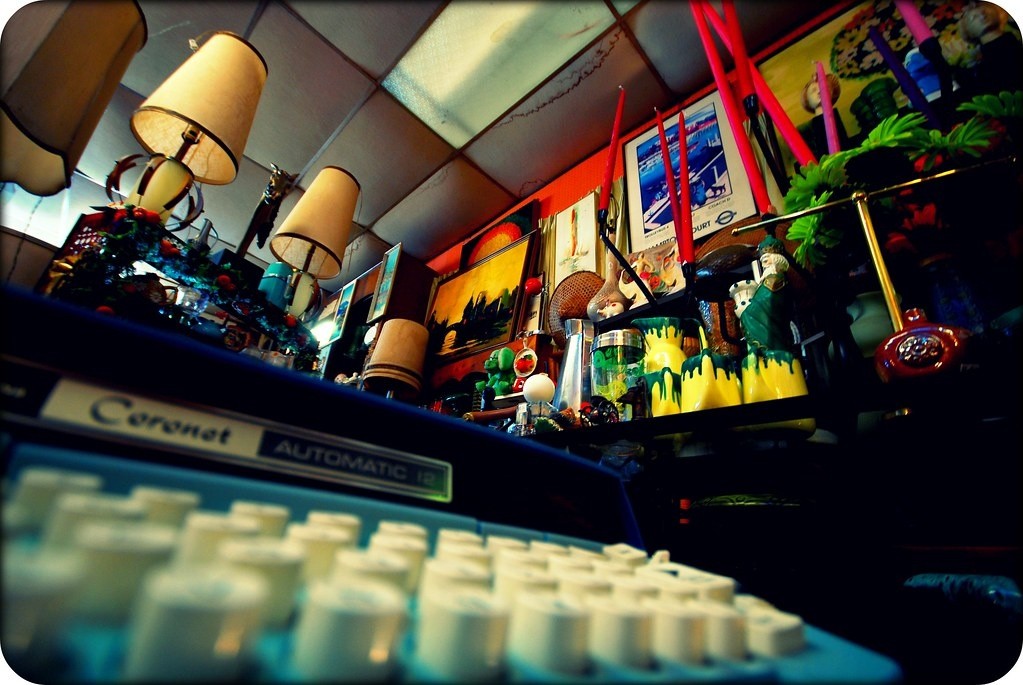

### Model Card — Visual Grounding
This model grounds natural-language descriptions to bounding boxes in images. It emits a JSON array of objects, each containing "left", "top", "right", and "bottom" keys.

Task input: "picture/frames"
[
  {"left": 366, "top": 242, "right": 403, "bottom": 323},
  {"left": 424, "top": 227, "right": 539, "bottom": 368},
  {"left": 555, "top": 191, "right": 601, "bottom": 292},
  {"left": 327, "top": 279, "right": 358, "bottom": 344},
  {"left": 754, "top": 0, "right": 1023, "bottom": 189},
  {"left": 622, "top": 81, "right": 761, "bottom": 250},
  {"left": 458, "top": 198, "right": 541, "bottom": 340}
]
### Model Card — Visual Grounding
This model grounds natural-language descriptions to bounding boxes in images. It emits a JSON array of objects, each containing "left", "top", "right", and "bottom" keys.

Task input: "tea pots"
[{"left": 121, "top": 272, "right": 178, "bottom": 309}]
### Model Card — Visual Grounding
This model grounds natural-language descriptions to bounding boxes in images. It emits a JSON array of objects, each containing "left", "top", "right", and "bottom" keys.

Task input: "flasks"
[{"left": 257, "top": 261, "right": 294, "bottom": 311}]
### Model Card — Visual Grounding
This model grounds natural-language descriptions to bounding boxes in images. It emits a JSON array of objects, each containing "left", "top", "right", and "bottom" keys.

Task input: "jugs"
[{"left": 188, "top": 218, "right": 218, "bottom": 256}]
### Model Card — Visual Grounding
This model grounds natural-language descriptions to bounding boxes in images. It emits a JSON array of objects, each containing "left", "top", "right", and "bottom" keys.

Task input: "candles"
[{"left": 594, "top": 0, "right": 934, "bottom": 264}]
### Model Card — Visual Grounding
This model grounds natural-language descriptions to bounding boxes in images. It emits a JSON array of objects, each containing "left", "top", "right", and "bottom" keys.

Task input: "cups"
[{"left": 591, "top": 330, "right": 643, "bottom": 421}]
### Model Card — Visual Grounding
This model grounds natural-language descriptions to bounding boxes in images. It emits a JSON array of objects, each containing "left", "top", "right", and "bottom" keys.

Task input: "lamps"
[
  {"left": 0, "top": 0, "right": 148, "bottom": 197},
  {"left": 269, "top": 166, "right": 360, "bottom": 316},
  {"left": 363, "top": 318, "right": 429, "bottom": 400},
  {"left": 103, "top": 28, "right": 268, "bottom": 232}
]
